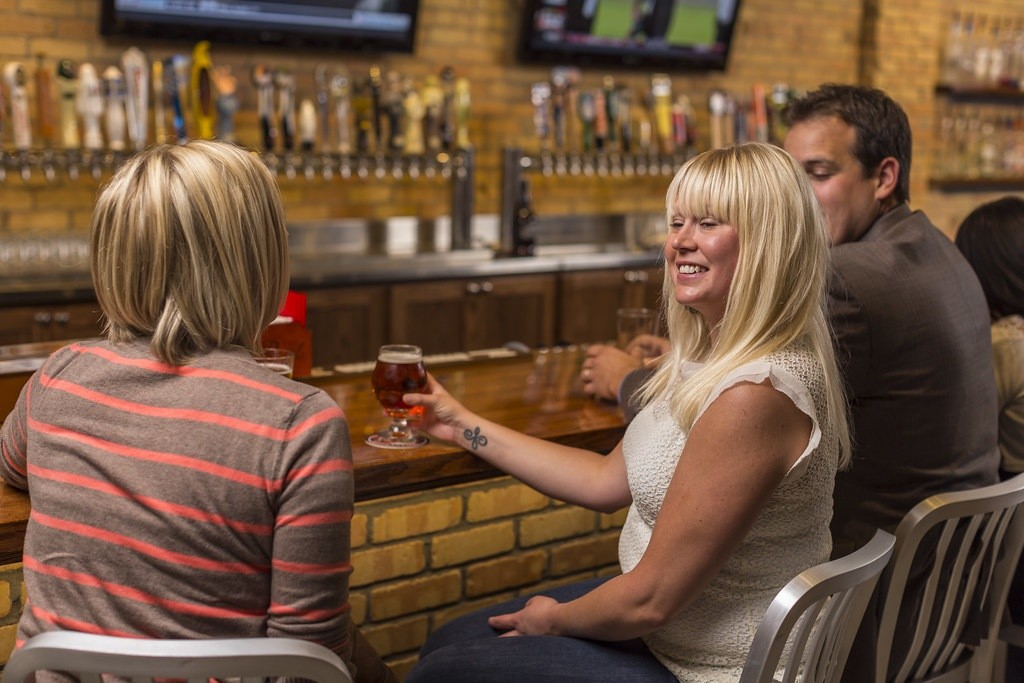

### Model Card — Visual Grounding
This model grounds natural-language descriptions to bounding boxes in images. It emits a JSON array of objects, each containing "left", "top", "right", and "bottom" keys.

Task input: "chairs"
[
  {"left": 847, "top": 472, "right": 1024, "bottom": 683},
  {"left": 733, "top": 525, "right": 899, "bottom": 683}
]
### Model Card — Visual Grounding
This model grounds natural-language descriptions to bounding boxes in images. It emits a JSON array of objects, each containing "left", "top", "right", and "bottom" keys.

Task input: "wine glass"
[{"left": 365, "top": 344, "right": 429, "bottom": 450}]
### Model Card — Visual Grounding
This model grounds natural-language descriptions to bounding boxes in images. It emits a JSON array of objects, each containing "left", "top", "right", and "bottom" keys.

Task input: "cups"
[
  {"left": 253, "top": 347, "right": 294, "bottom": 380},
  {"left": 618, "top": 309, "right": 658, "bottom": 362}
]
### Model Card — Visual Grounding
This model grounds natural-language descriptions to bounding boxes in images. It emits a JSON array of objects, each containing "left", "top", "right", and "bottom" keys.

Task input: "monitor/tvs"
[
  {"left": 101, "top": 0, "right": 419, "bottom": 57},
  {"left": 516, "top": 1, "right": 740, "bottom": 71}
]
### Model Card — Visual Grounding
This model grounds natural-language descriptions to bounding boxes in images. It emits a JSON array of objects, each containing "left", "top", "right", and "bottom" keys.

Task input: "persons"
[
  {"left": 0, "top": 141, "right": 400, "bottom": 682},
  {"left": 401, "top": 141, "right": 853, "bottom": 682},
  {"left": 580, "top": 83, "right": 1003, "bottom": 682},
  {"left": 953, "top": 195, "right": 1023, "bottom": 632}
]
